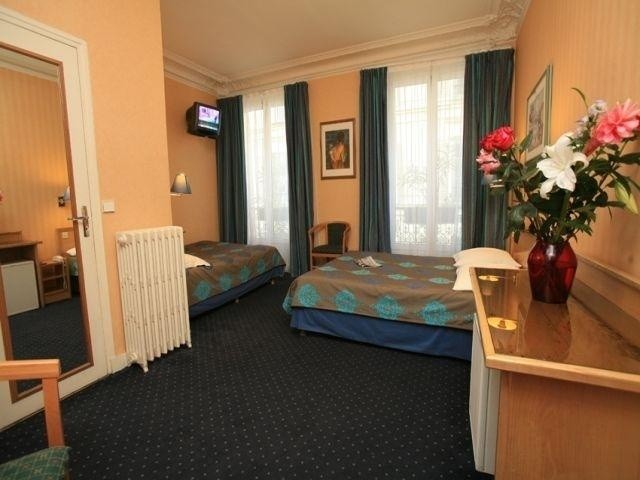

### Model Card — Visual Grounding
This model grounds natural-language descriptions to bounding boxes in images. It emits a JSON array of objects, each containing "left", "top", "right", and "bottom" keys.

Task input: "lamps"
[{"left": 168, "top": 171, "right": 192, "bottom": 198}]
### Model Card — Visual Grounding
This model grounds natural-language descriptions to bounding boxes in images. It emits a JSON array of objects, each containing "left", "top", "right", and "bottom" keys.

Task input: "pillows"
[
  {"left": 452, "top": 245, "right": 522, "bottom": 268},
  {"left": 450, "top": 261, "right": 519, "bottom": 291}
]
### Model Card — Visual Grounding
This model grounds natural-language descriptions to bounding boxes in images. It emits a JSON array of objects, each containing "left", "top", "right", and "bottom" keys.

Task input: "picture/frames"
[
  {"left": 525, "top": 62, "right": 554, "bottom": 166},
  {"left": 319, "top": 118, "right": 356, "bottom": 179}
]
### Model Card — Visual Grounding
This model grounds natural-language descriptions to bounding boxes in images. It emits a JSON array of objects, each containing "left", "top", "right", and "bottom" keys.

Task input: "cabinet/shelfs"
[{"left": 38, "top": 257, "right": 72, "bottom": 305}]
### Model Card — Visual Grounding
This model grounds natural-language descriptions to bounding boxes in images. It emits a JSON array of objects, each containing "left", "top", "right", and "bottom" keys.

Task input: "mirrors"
[{"left": 1, "top": 41, "right": 95, "bottom": 403}]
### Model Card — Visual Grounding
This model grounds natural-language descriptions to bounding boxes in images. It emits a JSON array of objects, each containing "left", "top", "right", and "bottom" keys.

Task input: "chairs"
[
  {"left": 308, "top": 221, "right": 351, "bottom": 273},
  {"left": 1, "top": 359, "right": 75, "bottom": 480}
]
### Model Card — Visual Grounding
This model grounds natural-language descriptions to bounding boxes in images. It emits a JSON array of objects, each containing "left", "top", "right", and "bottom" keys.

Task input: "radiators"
[{"left": 115, "top": 225, "right": 193, "bottom": 374}]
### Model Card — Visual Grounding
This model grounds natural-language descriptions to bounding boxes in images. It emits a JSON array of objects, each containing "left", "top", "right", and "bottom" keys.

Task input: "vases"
[{"left": 527, "top": 240, "right": 577, "bottom": 305}]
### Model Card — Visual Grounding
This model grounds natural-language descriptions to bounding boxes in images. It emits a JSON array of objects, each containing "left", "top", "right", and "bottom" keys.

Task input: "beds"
[
  {"left": 58, "top": 227, "right": 79, "bottom": 280},
  {"left": 282, "top": 230, "right": 544, "bottom": 361},
  {"left": 183, "top": 239, "right": 287, "bottom": 319}
]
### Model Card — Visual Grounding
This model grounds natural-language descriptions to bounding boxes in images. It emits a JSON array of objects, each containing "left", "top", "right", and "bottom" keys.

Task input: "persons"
[{"left": 329, "top": 132, "right": 348, "bottom": 169}]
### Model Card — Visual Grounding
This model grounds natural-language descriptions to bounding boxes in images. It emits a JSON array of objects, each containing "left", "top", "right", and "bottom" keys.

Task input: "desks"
[
  {"left": 468, "top": 263, "right": 640, "bottom": 479},
  {"left": 0, "top": 238, "right": 46, "bottom": 318}
]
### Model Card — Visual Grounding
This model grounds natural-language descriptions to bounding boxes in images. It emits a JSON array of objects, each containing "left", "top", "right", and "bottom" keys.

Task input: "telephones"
[{"left": 52, "top": 255, "right": 63, "bottom": 263}]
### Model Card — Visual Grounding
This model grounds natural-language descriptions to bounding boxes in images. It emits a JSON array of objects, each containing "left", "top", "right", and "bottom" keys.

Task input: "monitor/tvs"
[{"left": 185, "top": 102, "right": 222, "bottom": 140}]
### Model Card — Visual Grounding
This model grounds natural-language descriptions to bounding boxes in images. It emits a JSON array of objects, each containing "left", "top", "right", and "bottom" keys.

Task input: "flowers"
[{"left": 474, "top": 86, "right": 639, "bottom": 294}]
